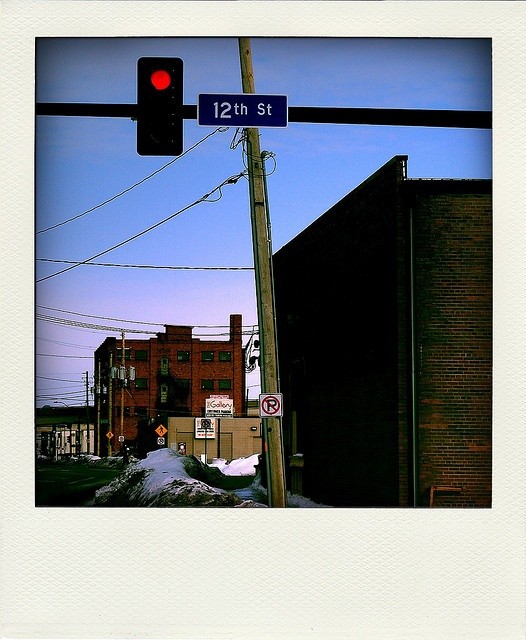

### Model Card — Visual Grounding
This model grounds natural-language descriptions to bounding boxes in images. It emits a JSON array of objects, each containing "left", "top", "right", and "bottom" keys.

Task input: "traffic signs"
[{"left": 138, "top": 57, "right": 184, "bottom": 156}]
